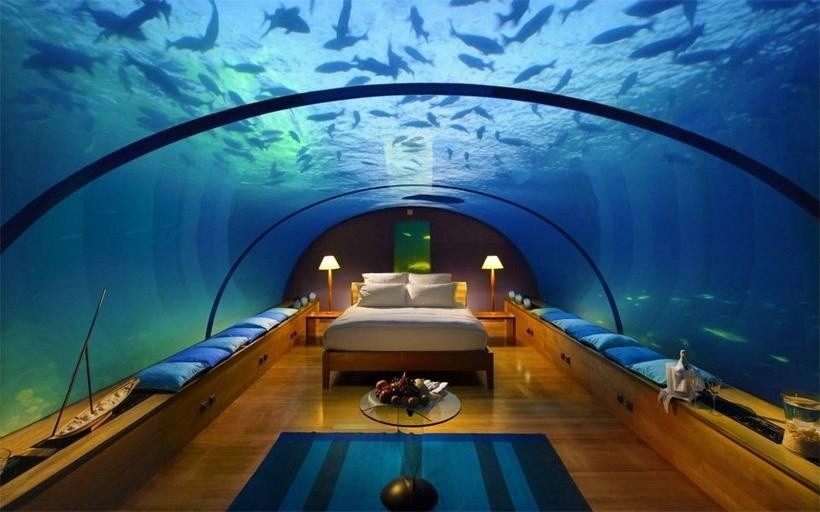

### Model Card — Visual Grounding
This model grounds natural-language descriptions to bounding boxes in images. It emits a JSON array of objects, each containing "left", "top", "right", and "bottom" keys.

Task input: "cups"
[
  {"left": 0, "top": 445, "right": 11, "bottom": 475},
  {"left": 781, "top": 395, "right": 820, "bottom": 423}
]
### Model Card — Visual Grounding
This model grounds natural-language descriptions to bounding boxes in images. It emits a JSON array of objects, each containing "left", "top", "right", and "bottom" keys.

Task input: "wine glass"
[{"left": 706, "top": 376, "right": 723, "bottom": 418}]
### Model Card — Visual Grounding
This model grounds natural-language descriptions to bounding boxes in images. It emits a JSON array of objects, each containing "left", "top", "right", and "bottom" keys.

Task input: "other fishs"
[
  {"left": 1, "top": 1, "right": 820, "bottom": 193},
  {"left": 704, "top": 325, "right": 748, "bottom": 344},
  {"left": 620, "top": 291, "right": 784, "bottom": 316},
  {"left": 767, "top": 353, "right": 789, "bottom": 364}
]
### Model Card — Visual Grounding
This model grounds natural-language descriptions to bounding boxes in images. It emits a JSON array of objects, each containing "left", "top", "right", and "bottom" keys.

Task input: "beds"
[{"left": 322, "top": 273, "right": 499, "bottom": 389}]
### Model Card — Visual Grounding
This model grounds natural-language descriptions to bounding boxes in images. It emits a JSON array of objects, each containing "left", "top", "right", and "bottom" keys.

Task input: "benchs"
[
  {"left": 20, "top": 303, "right": 317, "bottom": 512},
  {"left": 501, "top": 299, "right": 820, "bottom": 511}
]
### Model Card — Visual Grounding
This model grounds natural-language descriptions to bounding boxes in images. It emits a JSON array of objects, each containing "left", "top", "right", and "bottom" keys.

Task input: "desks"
[
  {"left": 357, "top": 382, "right": 463, "bottom": 512},
  {"left": 305, "top": 310, "right": 343, "bottom": 346},
  {"left": 474, "top": 312, "right": 517, "bottom": 346}
]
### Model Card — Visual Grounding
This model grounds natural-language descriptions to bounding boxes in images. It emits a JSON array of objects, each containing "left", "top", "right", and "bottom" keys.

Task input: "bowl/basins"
[{"left": 781, "top": 428, "right": 820, "bottom": 458}]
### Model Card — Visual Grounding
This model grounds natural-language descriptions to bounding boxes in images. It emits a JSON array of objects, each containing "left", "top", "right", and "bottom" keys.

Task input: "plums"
[
  {"left": 374, "top": 380, "right": 417, "bottom": 406},
  {"left": 421, "top": 395, "right": 429, "bottom": 405}
]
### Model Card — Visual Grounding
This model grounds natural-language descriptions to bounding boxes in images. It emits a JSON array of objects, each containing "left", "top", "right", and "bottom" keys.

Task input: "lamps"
[
  {"left": 318, "top": 255, "right": 341, "bottom": 313},
  {"left": 481, "top": 255, "right": 505, "bottom": 314}
]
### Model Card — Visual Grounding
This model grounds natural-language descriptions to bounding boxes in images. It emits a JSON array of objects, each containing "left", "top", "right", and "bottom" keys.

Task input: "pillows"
[
  {"left": 531, "top": 306, "right": 722, "bottom": 387},
  {"left": 406, "top": 282, "right": 459, "bottom": 308},
  {"left": 129, "top": 306, "right": 299, "bottom": 391},
  {"left": 406, "top": 272, "right": 454, "bottom": 283},
  {"left": 356, "top": 283, "right": 407, "bottom": 307},
  {"left": 362, "top": 270, "right": 408, "bottom": 284}
]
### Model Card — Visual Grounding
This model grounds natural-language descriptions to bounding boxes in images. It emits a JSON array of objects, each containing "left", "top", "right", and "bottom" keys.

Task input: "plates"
[{"left": 367, "top": 381, "right": 449, "bottom": 407}]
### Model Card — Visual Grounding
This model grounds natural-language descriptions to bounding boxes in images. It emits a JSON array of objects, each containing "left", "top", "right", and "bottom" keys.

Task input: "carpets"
[{"left": 226, "top": 429, "right": 594, "bottom": 512}]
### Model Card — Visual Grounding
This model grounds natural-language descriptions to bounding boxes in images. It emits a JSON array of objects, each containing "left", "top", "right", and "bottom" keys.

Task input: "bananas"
[{"left": 414, "top": 378, "right": 441, "bottom": 399}]
